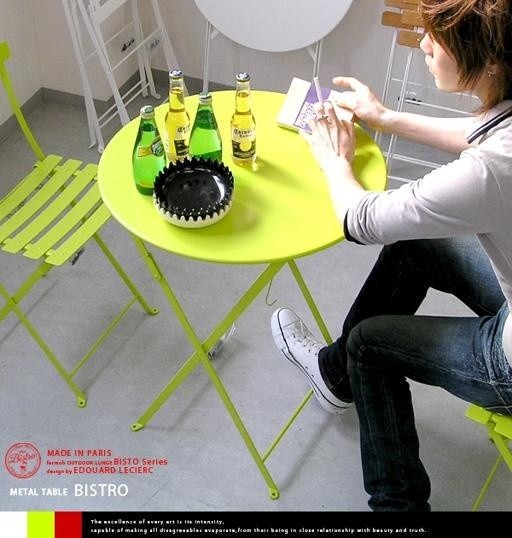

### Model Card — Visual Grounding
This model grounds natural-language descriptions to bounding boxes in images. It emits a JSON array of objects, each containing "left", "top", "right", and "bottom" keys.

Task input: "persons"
[{"left": 270, "top": 1, "right": 512, "bottom": 513}]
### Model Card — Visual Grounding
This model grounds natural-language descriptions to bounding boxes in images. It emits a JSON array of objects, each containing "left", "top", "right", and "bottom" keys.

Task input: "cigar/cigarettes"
[{"left": 314, "top": 77, "right": 325, "bottom": 108}]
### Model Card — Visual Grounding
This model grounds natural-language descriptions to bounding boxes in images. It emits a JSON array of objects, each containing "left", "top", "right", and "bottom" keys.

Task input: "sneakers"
[{"left": 271, "top": 306, "right": 352, "bottom": 416}]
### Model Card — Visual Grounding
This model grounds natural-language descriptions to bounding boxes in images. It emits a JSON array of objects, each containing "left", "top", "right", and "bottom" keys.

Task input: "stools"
[{"left": 465, "top": 403, "right": 512, "bottom": 512}]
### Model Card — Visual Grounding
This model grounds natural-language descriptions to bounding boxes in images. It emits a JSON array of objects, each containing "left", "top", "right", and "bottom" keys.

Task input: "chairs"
[{"left": 1, "top": 48, "right": 159, "bottom": 405}]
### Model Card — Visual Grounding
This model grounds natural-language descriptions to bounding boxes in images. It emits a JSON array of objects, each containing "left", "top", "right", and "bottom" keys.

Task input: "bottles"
[
  {"left": 186, "top": 90, "right": 224, "bottom": 165},
  {"left": 163, "top": 69, "right": 192, "bottom": 163},
  {"left": 131, "top": 104, "right": 168, "bottom": 196},
  {"left": 230, "top": 72, "right": 257, "bottom": 167}
]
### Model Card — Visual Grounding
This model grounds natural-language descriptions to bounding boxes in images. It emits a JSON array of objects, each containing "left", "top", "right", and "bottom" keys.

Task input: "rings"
[{"left": 316, "top": 113, "right": 327, "bottom": 120}]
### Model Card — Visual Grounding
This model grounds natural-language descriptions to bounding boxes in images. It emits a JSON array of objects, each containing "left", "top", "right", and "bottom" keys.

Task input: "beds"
[{"left": 96, "top": 90, "right": 390, "bottom": 499}]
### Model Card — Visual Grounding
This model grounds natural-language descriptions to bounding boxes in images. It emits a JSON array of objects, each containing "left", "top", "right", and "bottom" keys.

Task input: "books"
[{"left": 278, "top": 77, "right": 356, "bottom": 136}]
